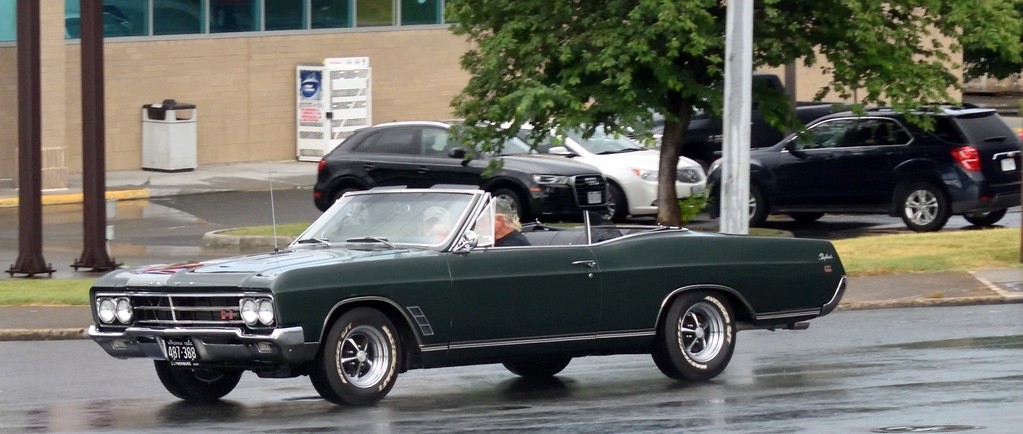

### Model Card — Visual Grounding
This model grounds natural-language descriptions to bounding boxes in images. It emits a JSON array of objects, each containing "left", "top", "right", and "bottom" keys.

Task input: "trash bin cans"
[{"left": 139, "top": 98, "right": 197, "bottom": 171}]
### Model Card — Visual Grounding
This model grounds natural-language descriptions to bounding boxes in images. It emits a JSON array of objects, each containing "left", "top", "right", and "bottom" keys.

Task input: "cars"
[
  {"left": 313, "top": 118, "right": 616, "bottom": 221},
  {"left": 447, "top": 117, "right": 707, "bottom": 221}
]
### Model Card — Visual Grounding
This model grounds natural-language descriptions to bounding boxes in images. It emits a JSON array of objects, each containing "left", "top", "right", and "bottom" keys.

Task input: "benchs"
[{"left": 522, "top": 231, "right": 598, "bottom": 246}]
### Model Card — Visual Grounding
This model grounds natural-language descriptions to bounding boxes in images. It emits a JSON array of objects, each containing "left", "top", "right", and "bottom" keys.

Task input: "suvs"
[{"left": 626, "top": 74, "right": 1023, "bottom": 232}]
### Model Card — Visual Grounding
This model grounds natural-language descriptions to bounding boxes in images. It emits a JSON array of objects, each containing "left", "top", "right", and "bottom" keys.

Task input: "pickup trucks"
[{"left": 89, "top": 184, "right": 847, "bottom": 406}]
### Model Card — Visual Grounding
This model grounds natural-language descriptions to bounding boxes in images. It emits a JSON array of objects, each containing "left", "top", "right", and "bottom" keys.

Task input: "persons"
[{"left": 423, "top": 195, "right": 532, "bottom": 247}]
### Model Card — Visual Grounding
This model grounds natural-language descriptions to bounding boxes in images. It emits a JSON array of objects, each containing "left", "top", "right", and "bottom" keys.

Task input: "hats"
[
  {"left": 494, "top": 197, "right": 511, "bottom": 215},
  {"left": 422, "top": 207, "right": 449, "bottom": 224}
]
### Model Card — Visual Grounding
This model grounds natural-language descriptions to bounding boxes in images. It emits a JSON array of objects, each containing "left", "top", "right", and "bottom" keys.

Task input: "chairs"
[
  {"left": 425, "top": 135, "right": 437, "bottom": 153},
  {"left": 874, "top": 124, "right": 888, "bottom": 143}
]
